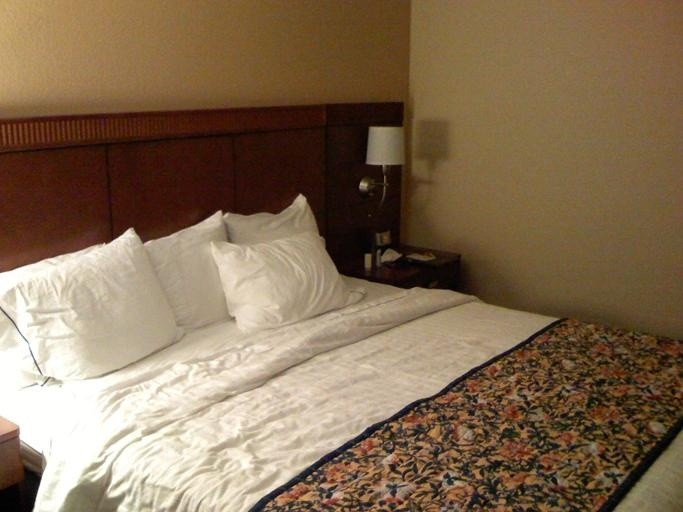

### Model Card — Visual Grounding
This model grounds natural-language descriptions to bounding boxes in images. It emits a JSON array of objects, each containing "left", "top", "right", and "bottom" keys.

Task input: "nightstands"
[
  {"left": 338, "top": 244, "right": 462, "bottom": 290},
  {"left": 0, "top": 419, "right": 24, "bottom": 489}
]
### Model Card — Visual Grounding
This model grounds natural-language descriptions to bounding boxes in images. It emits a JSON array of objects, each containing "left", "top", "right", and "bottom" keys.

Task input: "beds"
[{"left": 0, "top": 99, "right": 682, "bottom": 512}]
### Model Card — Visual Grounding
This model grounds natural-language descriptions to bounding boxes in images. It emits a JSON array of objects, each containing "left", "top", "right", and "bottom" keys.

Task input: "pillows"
[
  {"left": 223, "top": 193, "right": 325, "bottom": 246},
  {"left": 1, "top": 227, "right": 186, "bottom": 386},
  {"left": 210, "top": 232, "right": 364, "bottom": 336},
  {"left": 143, "top": 210, "right": 229, "bottom": 332}
]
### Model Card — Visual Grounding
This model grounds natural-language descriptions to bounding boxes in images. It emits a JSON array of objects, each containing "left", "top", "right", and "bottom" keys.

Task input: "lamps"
[{"left": 359, "top": 126, "right": 407, "bottom": 214}]
